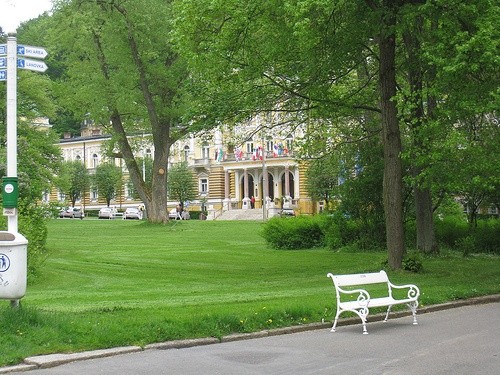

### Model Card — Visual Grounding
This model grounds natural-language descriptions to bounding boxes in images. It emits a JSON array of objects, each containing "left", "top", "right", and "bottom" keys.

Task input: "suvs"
[{"left": 58, "top": 206, "right": 84, "bottom": 219}]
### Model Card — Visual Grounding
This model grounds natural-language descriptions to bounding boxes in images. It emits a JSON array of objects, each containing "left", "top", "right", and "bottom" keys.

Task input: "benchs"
[{"left": 327, "top": 270, "right": 420, "bottom": 334}]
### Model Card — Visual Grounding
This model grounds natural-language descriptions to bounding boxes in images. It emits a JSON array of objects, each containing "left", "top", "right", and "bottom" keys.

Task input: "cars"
[
  {"left": 169, "top": 208, "right": 190, "bottom": 220},
  {"left": 123, "top": 208, "right": 141, "bottom": 220},
  {"left": 98, "top": 208, "right": 113, "bottom": 219}
]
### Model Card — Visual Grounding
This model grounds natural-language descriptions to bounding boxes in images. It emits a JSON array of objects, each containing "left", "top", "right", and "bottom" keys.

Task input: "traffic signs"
[
  {"left": 16, "top": 45, "right": 48, "bottom": 60},
  {"left": 17, "top": 58, "right": 49, "bottom": 74}
]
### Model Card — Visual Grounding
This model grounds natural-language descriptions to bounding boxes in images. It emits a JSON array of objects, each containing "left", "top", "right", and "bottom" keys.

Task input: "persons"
[
  {"left": 176, "top": 204, "right": 182, "bottom": 219},
  {"left": 251, "top": 195, "right": 255, "bottom": 208}
]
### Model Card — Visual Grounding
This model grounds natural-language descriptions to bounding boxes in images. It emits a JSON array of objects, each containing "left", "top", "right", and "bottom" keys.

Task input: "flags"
[{"left": 218, "top": 142, "right": 287, "bottom": 160}]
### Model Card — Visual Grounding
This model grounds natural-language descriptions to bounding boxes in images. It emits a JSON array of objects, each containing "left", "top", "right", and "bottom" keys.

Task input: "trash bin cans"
[{"left": 1, "top": 230, "right": 29, "bottom": 298}]
